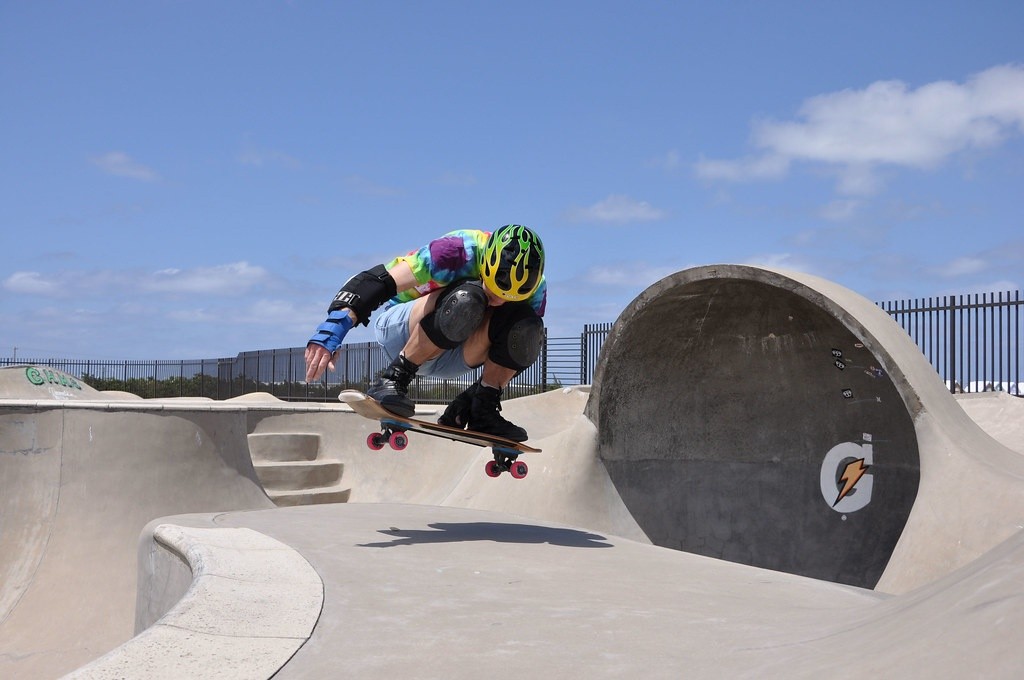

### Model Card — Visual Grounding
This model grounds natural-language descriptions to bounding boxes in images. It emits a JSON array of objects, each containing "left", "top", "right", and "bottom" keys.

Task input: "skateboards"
[{"left": 338, "top": 388, "right": 542, "bottom": 479}]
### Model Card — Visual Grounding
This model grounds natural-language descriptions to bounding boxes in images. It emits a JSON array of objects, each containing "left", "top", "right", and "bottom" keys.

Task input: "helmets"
[{"left": 478, "top": 223, "right": 544, "bottom": 301}]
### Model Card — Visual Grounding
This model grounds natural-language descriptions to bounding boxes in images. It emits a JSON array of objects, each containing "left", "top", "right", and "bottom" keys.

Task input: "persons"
[{"left": 300, "top": 224, "right": 549, "bottom": 445}]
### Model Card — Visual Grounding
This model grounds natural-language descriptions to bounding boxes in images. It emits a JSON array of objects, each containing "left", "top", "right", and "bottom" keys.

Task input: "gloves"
[{"left": 438, "top": 381, "right": 478, "bottom": 429}]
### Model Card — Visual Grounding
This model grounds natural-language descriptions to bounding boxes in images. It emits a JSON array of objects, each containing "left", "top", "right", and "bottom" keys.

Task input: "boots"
[
  {"left": 366, "top": 351, "right": 421, "bottom": 419},
  {"left": 466, "top": 379, "right": 529, "bottom": 442}
]
